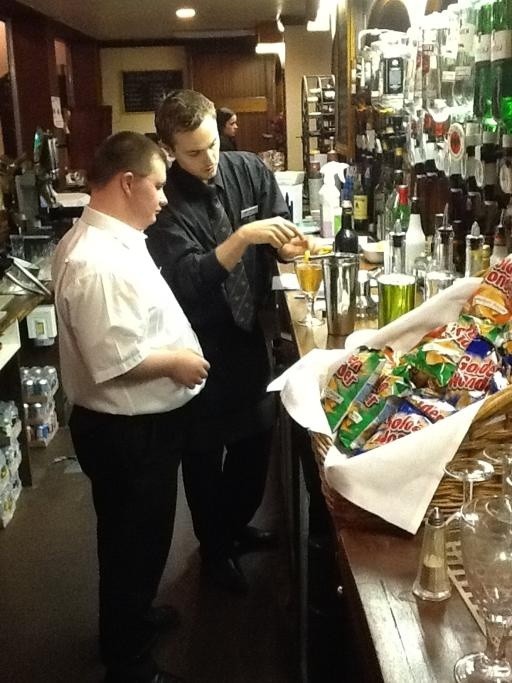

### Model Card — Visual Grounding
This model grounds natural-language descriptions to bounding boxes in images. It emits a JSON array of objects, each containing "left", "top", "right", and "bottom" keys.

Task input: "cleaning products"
[{"left": 318, "top": 161, "right": 350, "bottom": 237}]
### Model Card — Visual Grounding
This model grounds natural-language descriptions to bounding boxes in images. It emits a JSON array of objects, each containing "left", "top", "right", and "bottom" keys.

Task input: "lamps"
[
  {"left": 304, "top": 0, "right": 337, "bottom": 41},
  {"left": 254, "top": 21, "right": 287, "bottom": 54}
]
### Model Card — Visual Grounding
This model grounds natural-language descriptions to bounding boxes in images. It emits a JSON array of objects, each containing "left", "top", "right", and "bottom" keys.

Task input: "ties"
[{"left": 204, "top": 184, "right": 254, "bottom": 330}]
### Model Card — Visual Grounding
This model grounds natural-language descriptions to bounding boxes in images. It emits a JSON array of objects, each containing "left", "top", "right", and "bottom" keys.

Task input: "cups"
[{"left": 326, "top": 252, "right": 360, "bottom": 336}]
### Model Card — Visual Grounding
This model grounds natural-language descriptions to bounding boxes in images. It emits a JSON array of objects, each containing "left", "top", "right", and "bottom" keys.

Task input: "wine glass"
[
  {"left": 293, "top": 253, "right": 325, "bottom": 327},
  {"left": 444, "top": 442, "right": 512, "bottom": 683}
]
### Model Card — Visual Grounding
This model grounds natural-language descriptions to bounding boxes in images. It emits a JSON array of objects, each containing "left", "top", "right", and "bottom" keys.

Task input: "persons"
[
  {"left": 51, "top": 131, "right": 213, "bottom": 683},
  {"left": 217, "top": 108, "right": 238, "bottom": 152},
  {"left": 143, "top": 89, "right": 309, "bottom": 594}
]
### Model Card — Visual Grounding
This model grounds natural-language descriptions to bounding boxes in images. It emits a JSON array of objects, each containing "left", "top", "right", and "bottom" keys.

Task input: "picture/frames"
[{"left": 334, "top": 8, "right": 356, "bottom": 159}]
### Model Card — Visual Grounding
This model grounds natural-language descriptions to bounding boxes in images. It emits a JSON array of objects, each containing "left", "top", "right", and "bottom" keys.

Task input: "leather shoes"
[
  {"left": 150, "top": 605, "right": 179, "bottom": 640},
  {"left": 206, "top": 558, "right": 239, "bottom": 584},
  {"left": 119, "top": 667, "right": 185, "bottom": 683},
  {"left": 243, "top": 527, "right": 276, "bottom": 548}
]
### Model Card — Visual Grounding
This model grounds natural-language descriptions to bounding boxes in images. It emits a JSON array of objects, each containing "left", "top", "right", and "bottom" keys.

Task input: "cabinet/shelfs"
[{"left": 300, "top": 74, "right": 335, "bottom": 194}]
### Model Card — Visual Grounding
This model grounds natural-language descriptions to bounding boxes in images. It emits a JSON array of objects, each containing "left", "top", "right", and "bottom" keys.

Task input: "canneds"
[{"left": 19, "top": 366, "right": 60, "bottom": 441}]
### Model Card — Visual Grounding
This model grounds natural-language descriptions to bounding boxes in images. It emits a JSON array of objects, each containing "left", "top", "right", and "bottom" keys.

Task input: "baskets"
[{"left": 311, "top": 383, "right": 512, "bottom": 519}]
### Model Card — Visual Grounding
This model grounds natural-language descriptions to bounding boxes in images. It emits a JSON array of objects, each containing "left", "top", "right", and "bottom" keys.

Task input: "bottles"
[
  {"left": 307, "top": 160, "right": 325, "bottom": 217},
  {"left": 332, "top": 0, "right": 512, "bottom": 303},
  {"left": 411, "top": 504, "right": 452, "bottom": 602}
]
